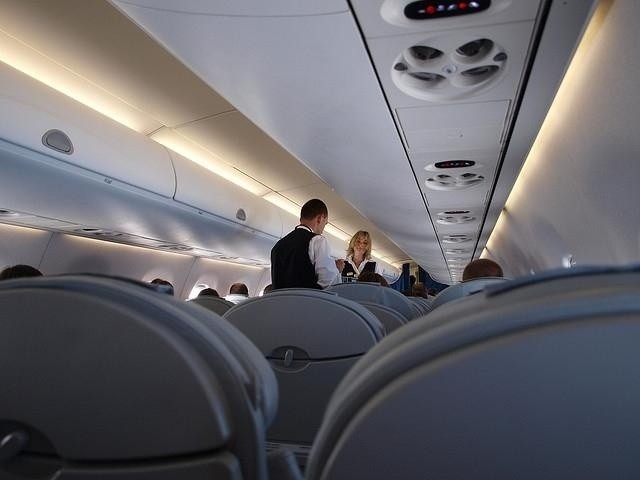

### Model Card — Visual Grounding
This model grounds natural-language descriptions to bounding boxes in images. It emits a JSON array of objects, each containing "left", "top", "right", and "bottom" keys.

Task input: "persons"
[
  {"left": 462, "top": 259, "right": 503, "bottom": 280},
  {"left": 0, "top": 265, "right": 43, "bottom": 281},
  {"left": 340, "top": 230, "right": 385, "bottom": 287},
  {"left": 151, "top": 279, "right": 174, "bottom": 295},
  {"left": 263, "top": 284, "right": 272, "bottom": 294},
  {"left": 357, "top": 271, "right": 390, "bottom": 288},
  {"left": 197, "top": 288, "right": 225, "bottom": 300},
  {"left": 271, "top": 199, "right": 345, "bottom": 290},
  {"left": 401, "top": 275, "right": 439, "bottom": 299},
  {"left": 230, "top": 283, "right": 248, "bottom": 297}
]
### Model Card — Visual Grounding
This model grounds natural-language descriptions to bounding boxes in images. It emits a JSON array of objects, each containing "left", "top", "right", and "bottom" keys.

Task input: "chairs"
[{"left": 0, "top": 263, "right": 640, "bottom": 480}]
